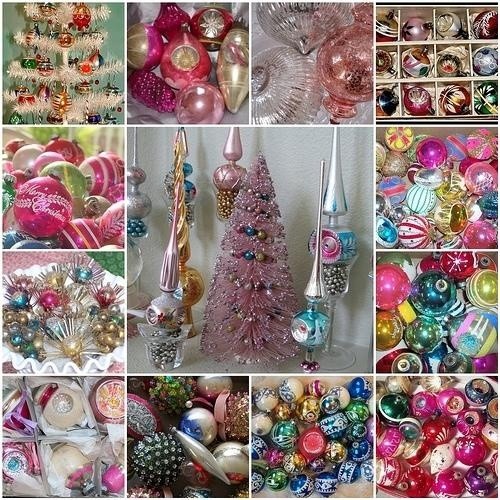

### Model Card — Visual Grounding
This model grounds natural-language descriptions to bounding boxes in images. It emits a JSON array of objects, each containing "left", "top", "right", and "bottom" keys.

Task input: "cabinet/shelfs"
[{"left": 376, "top": 5, "right": 498, "bottom": 119}]
